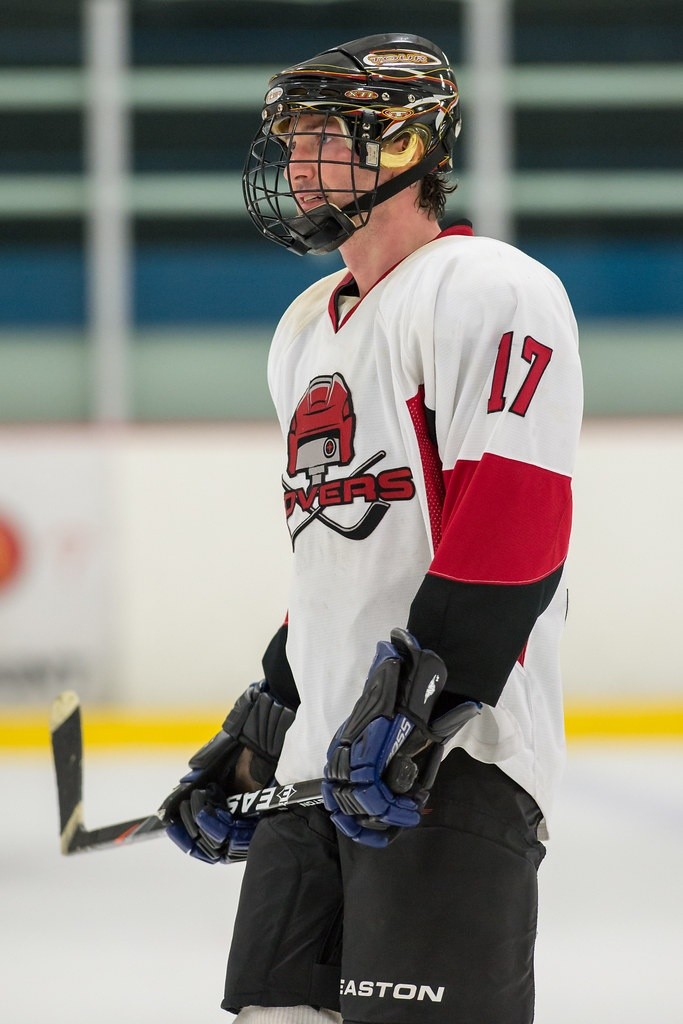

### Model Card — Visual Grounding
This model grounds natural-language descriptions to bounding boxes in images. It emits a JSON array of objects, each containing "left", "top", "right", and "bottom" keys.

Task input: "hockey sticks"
[{"left": 46, "top": 682, "right": 420, "bottom": 859}]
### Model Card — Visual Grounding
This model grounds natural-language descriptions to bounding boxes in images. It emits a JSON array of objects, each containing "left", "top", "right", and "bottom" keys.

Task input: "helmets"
[{"left": 260, "top": 30, "right": 458, "bottom": 171}]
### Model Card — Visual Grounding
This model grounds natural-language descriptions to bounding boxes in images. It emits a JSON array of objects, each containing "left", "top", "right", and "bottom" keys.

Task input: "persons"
[{"left": 160, "top": 32, "right": 586, "bottom": 1024}]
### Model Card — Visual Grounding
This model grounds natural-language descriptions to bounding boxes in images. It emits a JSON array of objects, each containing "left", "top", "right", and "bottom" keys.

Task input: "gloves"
[
  {"left": 321, "top": 626, "right": 481, "bottom": 849},
  {"left": 158, "top": 679, "right": 300, "bottom": 864}
]
